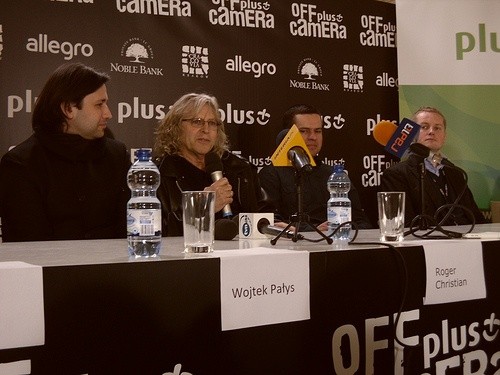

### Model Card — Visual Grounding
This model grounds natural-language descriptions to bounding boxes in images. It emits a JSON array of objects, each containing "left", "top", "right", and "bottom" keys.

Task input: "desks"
[{"left": 0, "top": 222, "right": 500, "bottom": 375}]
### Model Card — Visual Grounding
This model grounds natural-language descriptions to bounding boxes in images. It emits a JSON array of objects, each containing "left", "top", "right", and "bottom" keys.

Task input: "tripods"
[
  {"left": 403, "top": 157, "right": 463, "bottom": 240},
  {"left": 271, "top": 171, "right": 333, "bottom": 246}
]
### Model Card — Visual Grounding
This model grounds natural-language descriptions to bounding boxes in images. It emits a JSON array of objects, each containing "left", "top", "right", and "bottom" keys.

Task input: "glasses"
[{"left": 181, "top": 117, "right": 218, "bottom": 130}]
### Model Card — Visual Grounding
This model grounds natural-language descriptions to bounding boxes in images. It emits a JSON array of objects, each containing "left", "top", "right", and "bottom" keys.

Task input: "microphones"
[
  {"left": 270, "top": 123, "right": 317, "bottom": 173},
  {"left": 205, "top": 151, "right": 233, "bottom": 220},
  {"left": 214, "top": 219, "right": 238, "bottom": 240},
  {"left": 232, "top": 212, "right": 305, "bottom": 242},
  {"left": 373, "top": 117, "right": 455, "bottom": 170}
]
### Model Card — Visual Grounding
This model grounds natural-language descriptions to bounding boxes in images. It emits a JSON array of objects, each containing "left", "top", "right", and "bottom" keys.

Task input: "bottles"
[
  {"left": 126, "top": 149, "right": 161, "bottom": 257},
  {"left": 327, "top": 165, "right": 352, "bottom": 240}
]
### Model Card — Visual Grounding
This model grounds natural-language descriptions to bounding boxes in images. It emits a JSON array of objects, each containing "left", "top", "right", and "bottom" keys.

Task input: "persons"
[
  {"left": 260, "top": 105, "right": 375, "bottom": 233},
  {"left": 381, "top": 106, "right": 490, "bottom": 231},
  {"left": 0, "top": 59, "right": 169, "bottom": 241},
  {"left": 150, "top": 92, "right": 264, "bottom": 243}
]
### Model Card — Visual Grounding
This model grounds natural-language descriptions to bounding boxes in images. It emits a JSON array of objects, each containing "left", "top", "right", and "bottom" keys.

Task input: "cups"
[
  {"left": 377, "top": 191, "right": 405, "bottom": 244},
  {"left": 182, "top": 191, "right": 215, "bottom": 254}
]
